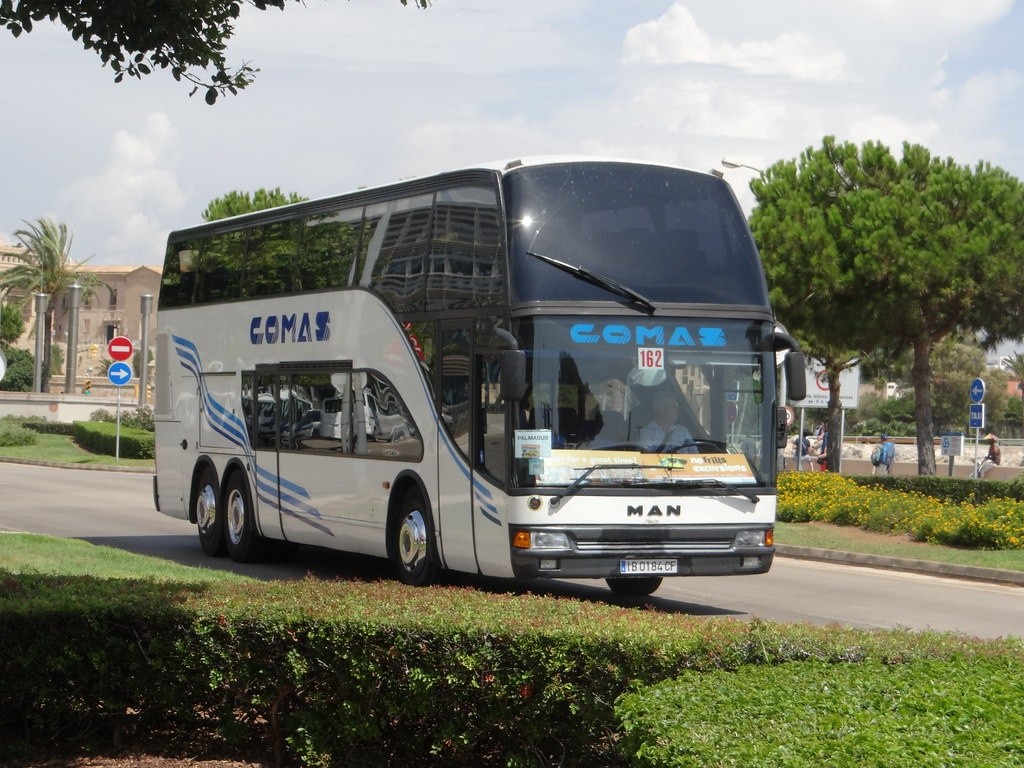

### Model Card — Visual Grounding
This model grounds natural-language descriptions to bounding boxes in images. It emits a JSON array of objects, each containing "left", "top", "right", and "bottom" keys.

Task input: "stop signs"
[{"left": 108, "top": 337, "right": 132, "bottom": 361}]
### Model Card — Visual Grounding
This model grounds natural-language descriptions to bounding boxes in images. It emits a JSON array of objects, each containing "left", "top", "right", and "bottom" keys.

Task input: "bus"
[
  {"left": 321, "top": 391, "right": 380, "bottom": 442},
  {"left": 153, "top": 153, "right": 809, "bottom": 597}
]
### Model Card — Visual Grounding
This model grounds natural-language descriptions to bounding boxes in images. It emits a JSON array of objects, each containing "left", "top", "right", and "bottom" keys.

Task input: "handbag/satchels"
[
  {"left": 817, "top": 457, "right": 825, "bottom": 464},
  {"left": 871, "top": 446, "right": 882, "bottom": 465}
]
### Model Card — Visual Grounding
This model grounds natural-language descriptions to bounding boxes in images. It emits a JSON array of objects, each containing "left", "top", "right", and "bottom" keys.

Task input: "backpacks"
[{"left": 883, "top": 444, "right": 895, "bottom": 465}]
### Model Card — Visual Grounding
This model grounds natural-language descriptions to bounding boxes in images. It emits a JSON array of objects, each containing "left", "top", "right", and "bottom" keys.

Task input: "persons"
[
  {"left": 82, "top": 380, "right": 91, "bottom": 396},
  {"left": 980, "top": 432, "right": 1001, "bottom": 479},
  {"left": 792, "top": 418, "right": 827, "bottom": 471},
  {"left": 872, "top": 434, "right": 893, "bottom": 475},
  {"left": 639, "top": 390, "right": 699, "bottom": 454}
]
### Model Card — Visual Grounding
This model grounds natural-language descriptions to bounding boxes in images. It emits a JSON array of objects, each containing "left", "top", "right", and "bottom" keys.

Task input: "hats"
[{"left": 983, "top": 433, "right": 998, "bottom": 440}]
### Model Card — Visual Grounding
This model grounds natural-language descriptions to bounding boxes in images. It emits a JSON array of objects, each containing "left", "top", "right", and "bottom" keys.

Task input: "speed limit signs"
[{"left": 786, "top": 407, "right": 795, "bottom": 428}]
